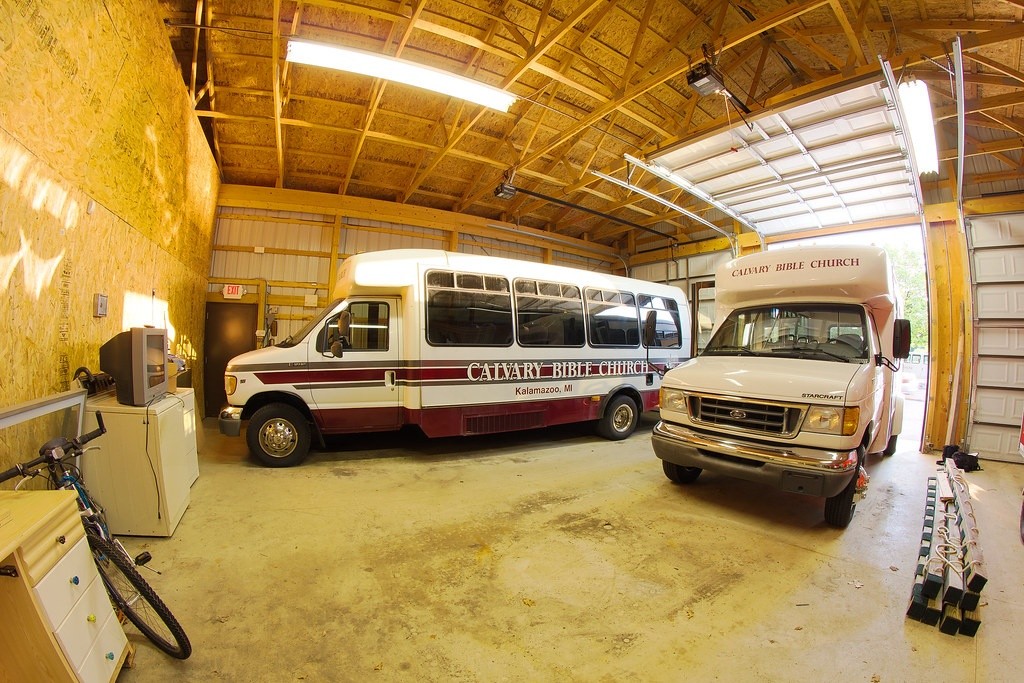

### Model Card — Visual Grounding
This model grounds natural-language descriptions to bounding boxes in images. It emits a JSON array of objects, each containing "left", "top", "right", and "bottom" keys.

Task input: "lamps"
[
  {"left": 898, "top": 74, "right": 939, "bottom": 176},
  {"left": 285, "top": 37, "right": 523, "bottom": 113}
]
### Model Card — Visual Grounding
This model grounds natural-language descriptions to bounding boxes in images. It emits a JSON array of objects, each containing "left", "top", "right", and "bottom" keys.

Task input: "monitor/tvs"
[{"left": 100, "top": 328, "right": 169, "bottom": 407}]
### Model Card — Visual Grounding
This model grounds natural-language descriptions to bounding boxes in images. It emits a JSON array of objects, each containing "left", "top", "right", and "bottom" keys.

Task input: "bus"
[
  {"left": 643, "top": 244, "right": 912, "bottom": 532},
  {"left": 219, "top": 248, "right": 693, "bottom": 469}
]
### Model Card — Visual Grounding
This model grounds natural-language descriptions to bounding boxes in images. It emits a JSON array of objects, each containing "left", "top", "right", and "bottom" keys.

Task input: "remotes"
[{"left": 144, "top": 325, "right": 155, "bottom": 328}]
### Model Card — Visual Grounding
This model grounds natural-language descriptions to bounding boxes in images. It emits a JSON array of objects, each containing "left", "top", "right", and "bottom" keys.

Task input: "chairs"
[
  {"left": 464, "top": 324, "right": 660, "bottom": 345},
  {"left": 747, "top": 334, "right": 863, "bottom": 357}
]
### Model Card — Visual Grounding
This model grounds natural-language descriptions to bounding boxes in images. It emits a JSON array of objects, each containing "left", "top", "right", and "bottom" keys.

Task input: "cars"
[{"left": 905, "top": 347, "right": 928, "bottom": 379}]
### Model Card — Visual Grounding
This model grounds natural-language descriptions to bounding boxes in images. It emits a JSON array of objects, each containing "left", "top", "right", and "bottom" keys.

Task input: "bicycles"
[{"left": 1, "top": 413, "right": 198, "bottom": 662}]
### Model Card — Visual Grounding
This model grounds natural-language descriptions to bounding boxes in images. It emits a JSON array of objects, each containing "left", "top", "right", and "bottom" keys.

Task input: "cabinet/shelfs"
[
  {"left": 71, "top": 388, "right": 199, "bottom": 537},
  {"left": 0, "top": 490, "right": 135, "bottom": 683}
]
